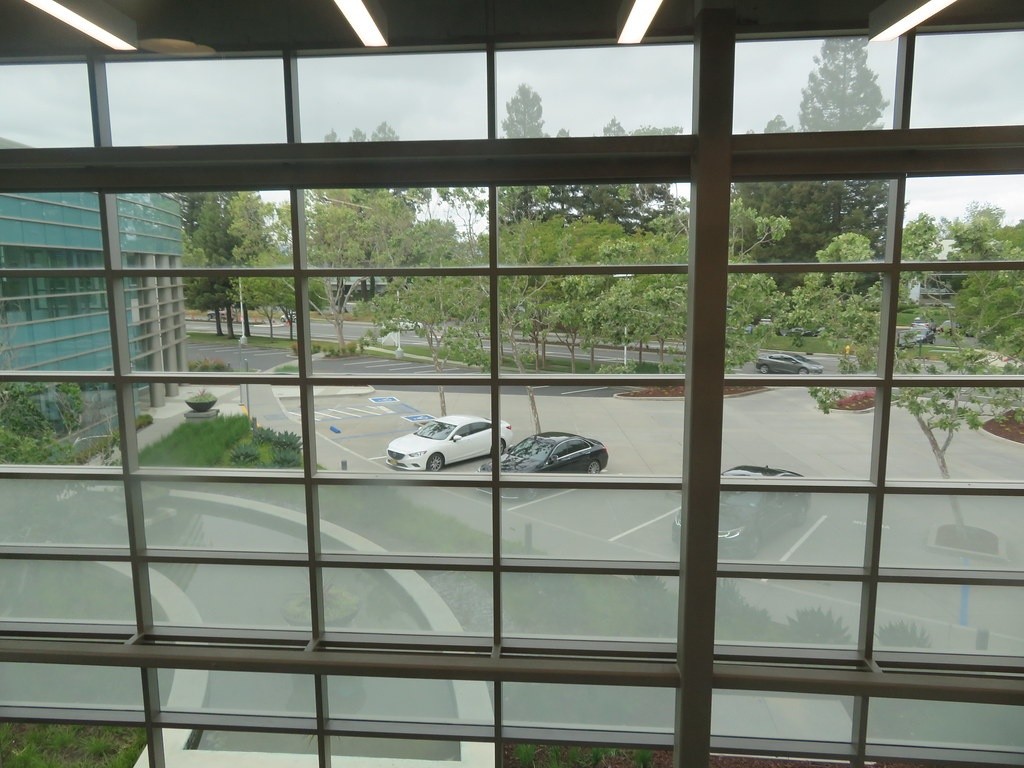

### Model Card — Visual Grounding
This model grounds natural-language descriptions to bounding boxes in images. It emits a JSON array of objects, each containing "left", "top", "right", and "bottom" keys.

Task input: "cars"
[
  {"left": 386, "top": 415, "right": 513, "bottom": 469},
  {"left": 755, "top": 352, "right": 824, "bottom": 376},
  {"left": 207, "top": 309, "right": 234, "bottom": 321},
  {"left": 910, "top": 315, "right": 961, "bottom": 345},
  {"left": 672, "top": 464, "right": 811, "bottom": 556},
  {"left": 744, "top": 316, "right": 819, "bottom": 339},
  {"left": 281, "top": 311, "right": 297, "bottom": 323},
  {"left": 474, "top": 432, "right": 608, "bottom": 504},
  {"left": 382, "top": 316, "right": 423, "bottom": 333}
]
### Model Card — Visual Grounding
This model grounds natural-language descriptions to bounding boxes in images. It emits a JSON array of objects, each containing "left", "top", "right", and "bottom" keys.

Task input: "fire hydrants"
[{"left": 845, "top": 345, "right": 851, "bottom": 354}]
